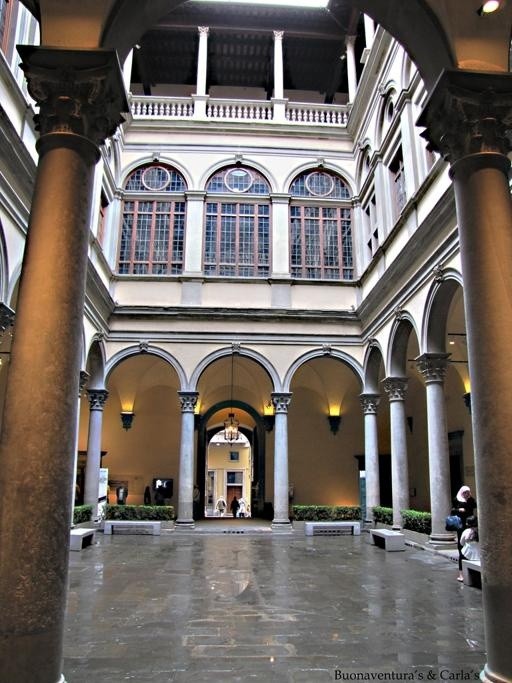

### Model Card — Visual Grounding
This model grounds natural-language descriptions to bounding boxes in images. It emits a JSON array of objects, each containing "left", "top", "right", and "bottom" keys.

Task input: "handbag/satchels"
[{"left": 445, "top": 515, "right": 462, "bottom": 531}]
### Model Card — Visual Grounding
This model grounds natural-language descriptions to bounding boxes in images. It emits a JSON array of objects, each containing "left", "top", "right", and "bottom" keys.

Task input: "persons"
[
  {"left": 75, "top": 483, "right": 80, "bottom": 506},
  {"left": 215, "top": 496, "right": 245, "bottom": 517},
  {"left": 445, "top": 485, "right": 482, "bottom": 582},
  {"left": 194, "top": 484, "right": 200, "bottom": 520},
  {"left": 144, "top": 486, "right": 165, "bottom": 506},
  {"left": 116, "top": 481, "right": 128, "bottom": 505}
]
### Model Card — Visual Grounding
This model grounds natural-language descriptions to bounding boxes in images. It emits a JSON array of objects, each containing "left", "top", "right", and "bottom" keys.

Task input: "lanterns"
[{"left": 224, "top": 413, "right": 239, "bottom": 445}]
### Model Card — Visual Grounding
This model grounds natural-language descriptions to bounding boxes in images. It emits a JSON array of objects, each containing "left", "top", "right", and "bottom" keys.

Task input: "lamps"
[
  {"left": 407, "top": 416, "right": 413, "bottom": 431},
  {"left": 463, "top": 385, "right": 471, "bottom": 415},
  {"left": 328, "top": 407, "right": 341, "bottom": 436},
  {"left": 121, "top": 402, "right": 134, "bottom": 432},
  {"left": 263, "top": 406, "right": 275, "bottom": 433},
  {"left": 223, "top": 352, "right": 239, "bottom": 447},
  {"left": 192, "top": 403, "right": 201, "bottom": 433}
]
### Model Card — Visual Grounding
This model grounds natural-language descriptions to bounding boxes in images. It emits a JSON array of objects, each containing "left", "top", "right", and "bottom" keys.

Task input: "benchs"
[
  {"left": 304, "top": 522, "right": 361, "bottom": 536},
  {"left": 369, "top": 528, "right": 405, "bottom": 551},
  {"left": 461, "top": 559, "right": 482, "bottom": 586},
  {"left": 70, "top": 528, "right": 96, "bottom": 551},
  {"left": 104, "top": 519, "right": 161, "bottom": 536}
]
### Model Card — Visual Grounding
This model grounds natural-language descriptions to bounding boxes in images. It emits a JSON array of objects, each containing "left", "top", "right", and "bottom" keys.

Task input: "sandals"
[{"left": 457, "top": 574, "right": 464, "bottom": 582}]
